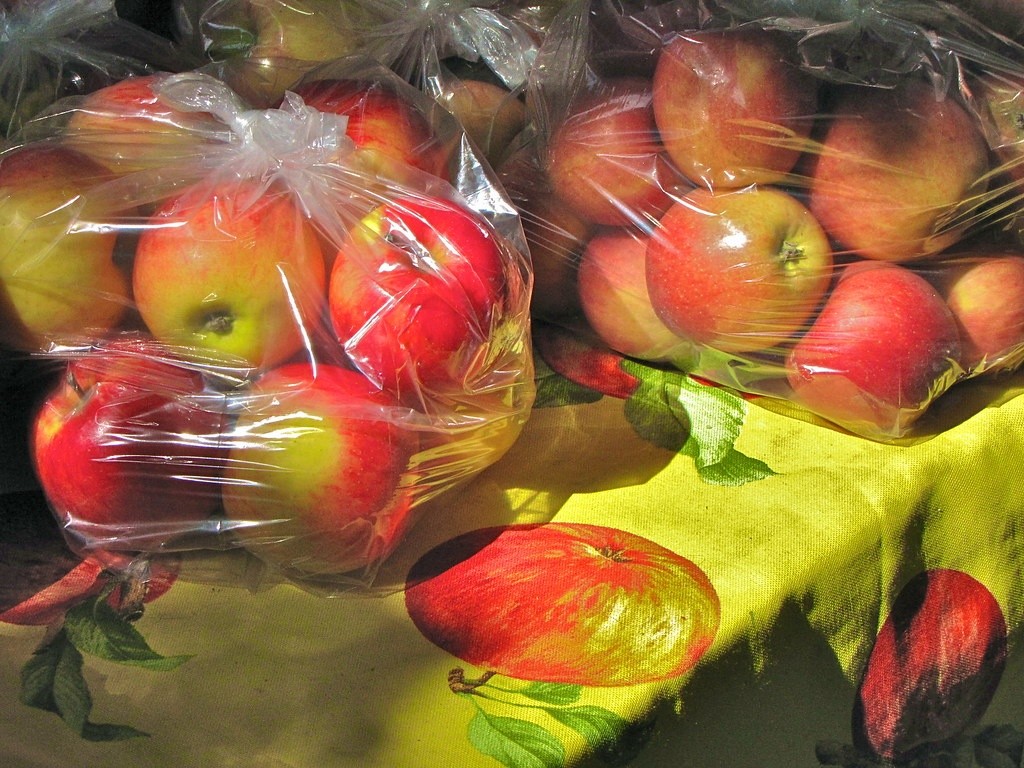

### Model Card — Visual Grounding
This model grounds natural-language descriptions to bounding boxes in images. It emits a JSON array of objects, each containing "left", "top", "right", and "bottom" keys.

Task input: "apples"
[{"left": 1, "top": 1, "right": 1024, "bottom": 574}]
